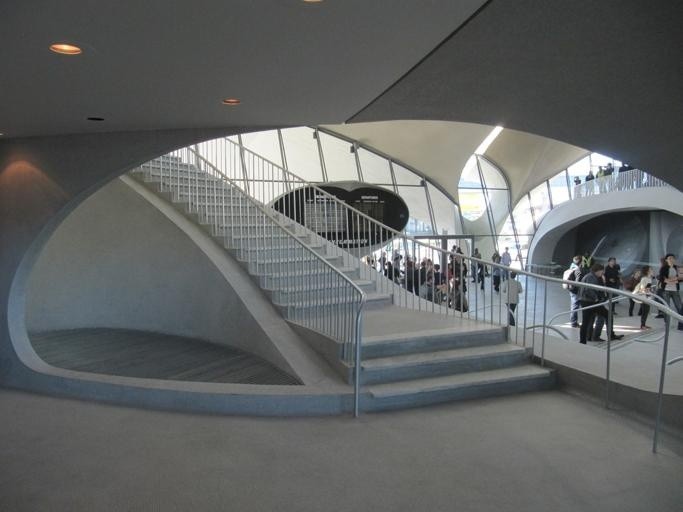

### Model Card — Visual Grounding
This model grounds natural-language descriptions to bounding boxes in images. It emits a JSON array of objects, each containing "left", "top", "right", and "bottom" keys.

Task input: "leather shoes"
[{"left": 611, "top": 334, "right": 624, "bottom": 340}]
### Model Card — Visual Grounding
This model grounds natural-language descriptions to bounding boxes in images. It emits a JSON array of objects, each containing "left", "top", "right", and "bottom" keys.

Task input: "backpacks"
[{"left": 562, "top": 267, "right": 577, "bottom": 289}]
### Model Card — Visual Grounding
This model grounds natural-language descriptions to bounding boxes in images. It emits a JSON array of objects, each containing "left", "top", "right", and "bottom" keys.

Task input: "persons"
[{"left": 574, "top": 163, "right": 632, "bottom": 196}]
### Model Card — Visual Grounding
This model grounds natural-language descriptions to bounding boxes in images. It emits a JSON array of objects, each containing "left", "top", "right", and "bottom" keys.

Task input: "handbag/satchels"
[{"left": 568, "top": 271, "right": 584, "bottom": 294}]
